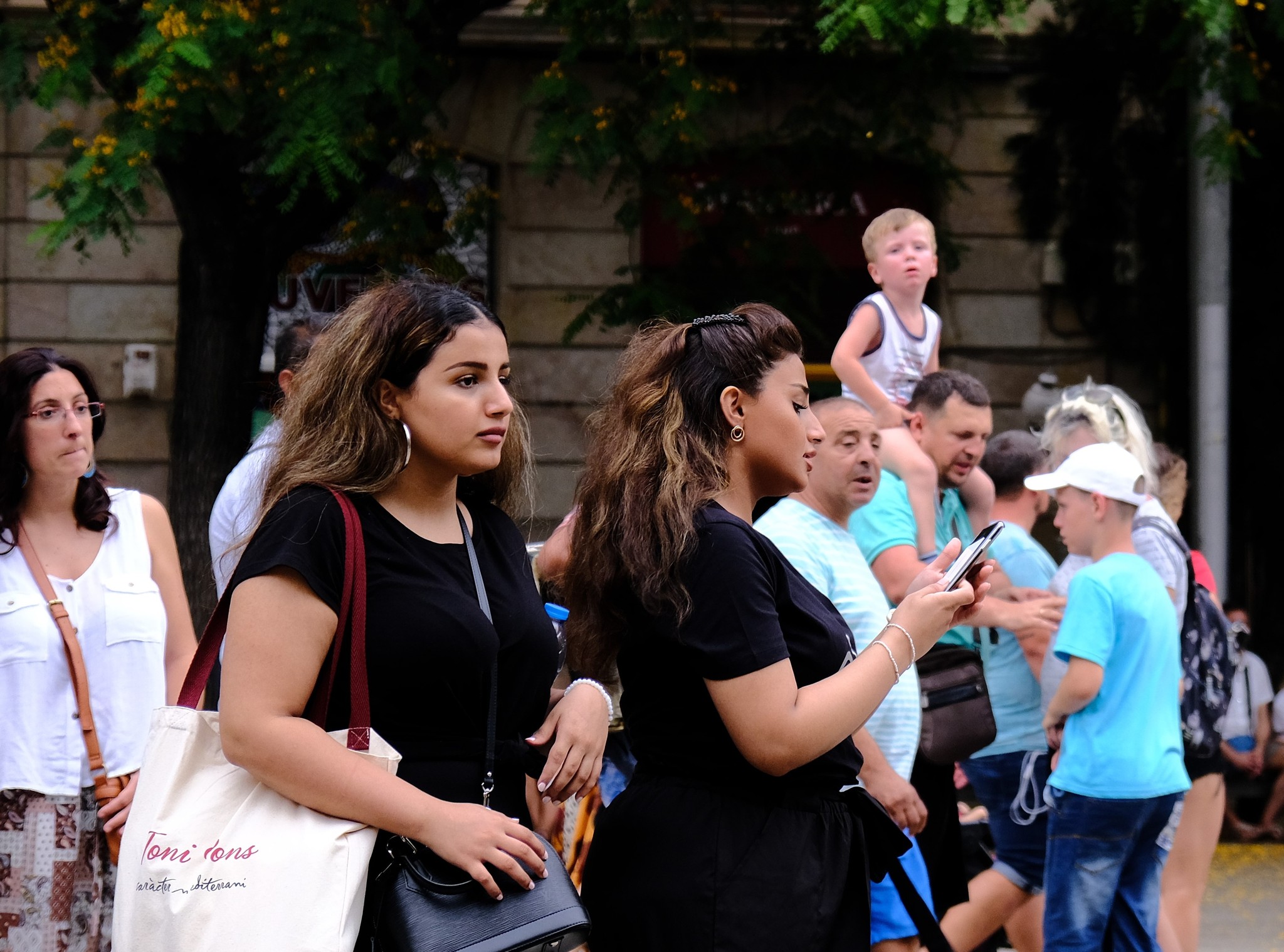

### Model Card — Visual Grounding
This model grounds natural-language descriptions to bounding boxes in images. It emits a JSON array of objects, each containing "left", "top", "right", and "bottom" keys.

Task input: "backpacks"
[{"left": 1131, "top": 516, "right": 1237, "bottom": 756}]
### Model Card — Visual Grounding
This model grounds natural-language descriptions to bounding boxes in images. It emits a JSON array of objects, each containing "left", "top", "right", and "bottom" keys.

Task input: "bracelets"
[
  {"left": 885, "top": 624, "right": 915, "bottom": 672},
  {"left": 870, "top": 641, "right": 900, "bottom": 688},
  {"left": 563, "top": 678, "right": 614, "bottom": 729}
]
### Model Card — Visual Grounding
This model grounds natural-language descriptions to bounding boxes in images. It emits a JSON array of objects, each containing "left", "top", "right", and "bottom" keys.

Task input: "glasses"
[{"left": 29, "top": 401, "right": 105, "bottom": 424}]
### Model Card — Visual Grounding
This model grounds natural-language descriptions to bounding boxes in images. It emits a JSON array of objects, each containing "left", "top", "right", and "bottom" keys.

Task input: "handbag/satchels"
[
  {"left": 374, "top": 835, "right": 591, "bottom": 952},
  {"left": 916, "top": 646, "right": 997, "bottom": 762},
  {"left": 111, "top": 702, "right": 402, "bottom": 952}
]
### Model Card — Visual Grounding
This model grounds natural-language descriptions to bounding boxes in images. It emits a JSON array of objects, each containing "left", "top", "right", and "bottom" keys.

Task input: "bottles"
[{"left": 545, "top": 602, "right": 570, "bottom": 688}]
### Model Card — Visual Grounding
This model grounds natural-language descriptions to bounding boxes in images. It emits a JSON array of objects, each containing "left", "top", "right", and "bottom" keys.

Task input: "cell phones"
[{"left": 941, "top": 521, "right": 1007, "bottom": 592}]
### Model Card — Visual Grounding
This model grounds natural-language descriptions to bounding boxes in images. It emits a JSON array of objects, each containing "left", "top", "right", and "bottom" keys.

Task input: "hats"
[{"left": 1023, "top": 441, "right": 1147, "bottom": 506}]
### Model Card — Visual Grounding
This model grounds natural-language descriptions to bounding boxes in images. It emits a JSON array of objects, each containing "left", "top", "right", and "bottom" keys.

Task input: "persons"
[
  {"left": 216, "top": 278, "right": 617, "bottom": 952},
  {"left": 207, "top": 309, "right": 340, "bottom": 683},
  {"left": 561, "top": 302, "right": 974, "bottom": 952},
  {"left": 1, "top": 347, "right": 206, "bottom": 952},
  {"left": 526, "top": 205, "right": 1283, "bottom": 951}
]
